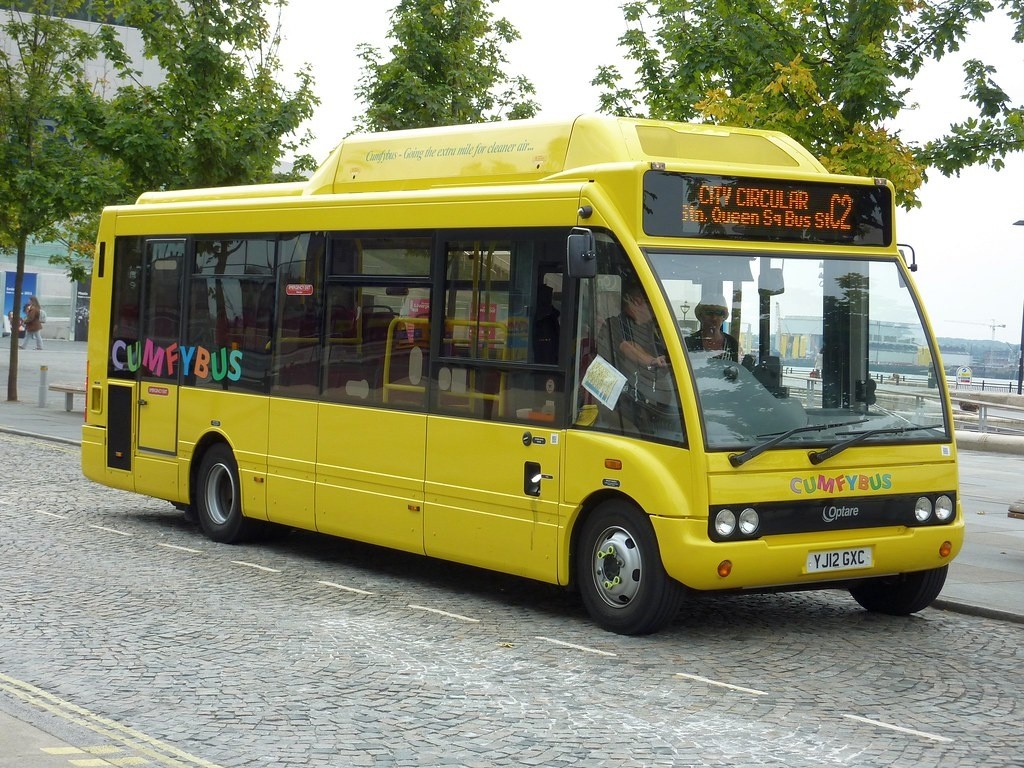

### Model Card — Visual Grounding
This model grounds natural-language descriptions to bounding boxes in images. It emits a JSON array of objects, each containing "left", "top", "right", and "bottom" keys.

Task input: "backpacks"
[{"left": 31, "top": 306, "right": 47, "bottom": 323}]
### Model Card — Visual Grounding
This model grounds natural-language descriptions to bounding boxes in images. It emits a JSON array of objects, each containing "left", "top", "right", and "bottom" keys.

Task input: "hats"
[{"left": 695, "top": 294, "right": 729, "bottom": 323}]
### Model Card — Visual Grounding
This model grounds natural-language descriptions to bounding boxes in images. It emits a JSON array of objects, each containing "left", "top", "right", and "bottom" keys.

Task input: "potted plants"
[{"left": 959, "top": 401, "right": 979, "bottom": 413}]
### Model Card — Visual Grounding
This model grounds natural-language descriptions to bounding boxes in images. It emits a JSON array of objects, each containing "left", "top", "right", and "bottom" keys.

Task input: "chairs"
[
  {"left": 582, "top": 338, "right": 647, "bottom": 434},
  {"left": 119, "top": 302, "right": 488, "bottom": 411}
]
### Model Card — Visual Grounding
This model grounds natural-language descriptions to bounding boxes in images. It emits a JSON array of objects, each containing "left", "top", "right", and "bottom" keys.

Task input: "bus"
[{"left": 80, "top": 112, "right": 969, "bottom": 637}]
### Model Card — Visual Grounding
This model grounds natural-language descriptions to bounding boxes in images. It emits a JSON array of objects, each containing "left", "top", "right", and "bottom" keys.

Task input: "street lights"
[{"left": 680, "top": 300, "right": 689, "bottom": 321}]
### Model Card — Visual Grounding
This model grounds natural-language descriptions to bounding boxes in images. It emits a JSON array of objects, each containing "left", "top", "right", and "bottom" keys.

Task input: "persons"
[
  {"left": 786, "top": 367, "right": 905, "bottom": 385},
  {"left": 20, "top": 296, "right": 44, "bottom": 351},
  {"left": 598, "top": 275, "right": 672, "bottom": 435},
  {"left": 682, "top": 294, "right": 742, "bottom": 383},
  {"left": 5, "top": 311, "right": 24, "bottom": 333}
]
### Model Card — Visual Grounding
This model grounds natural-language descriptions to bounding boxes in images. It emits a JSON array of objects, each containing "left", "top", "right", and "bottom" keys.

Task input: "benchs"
[{"left": 48, "top": 384, "right": 87, "bottom": 411}]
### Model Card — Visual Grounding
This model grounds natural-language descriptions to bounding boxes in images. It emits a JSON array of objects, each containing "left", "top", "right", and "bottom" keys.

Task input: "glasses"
[{"left": 701, "top": 308, "right": 725, "bottom": 316}]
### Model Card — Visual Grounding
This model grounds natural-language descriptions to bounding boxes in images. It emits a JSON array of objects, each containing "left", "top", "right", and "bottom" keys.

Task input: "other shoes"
[
  {"left": 19, "top": 345, "right": 26, "bottom": 350},
  {"left": 33, "top": 347, "right": 43, "bottom": 350}
]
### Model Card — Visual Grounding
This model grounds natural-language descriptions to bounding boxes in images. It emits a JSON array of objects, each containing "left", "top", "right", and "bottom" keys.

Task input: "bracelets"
[{"left": 648, "top": 359, "right": 655, "bottom": 370}]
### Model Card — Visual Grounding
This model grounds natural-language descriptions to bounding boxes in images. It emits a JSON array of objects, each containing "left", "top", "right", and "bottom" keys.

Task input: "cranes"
[{"left": 942, "top": 317, "right": 1006, "bottom": 364}]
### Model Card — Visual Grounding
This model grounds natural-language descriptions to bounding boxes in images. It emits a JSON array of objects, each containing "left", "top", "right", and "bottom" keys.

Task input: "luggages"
[{"left": 810, "top": 369, "right": 820, "bottom": 378}]
